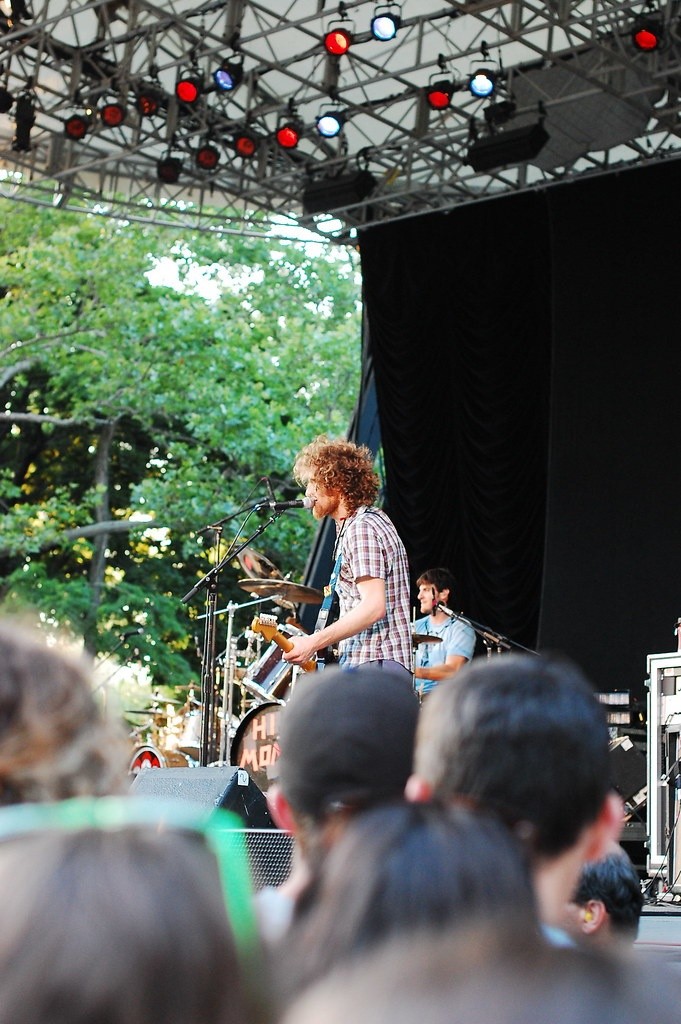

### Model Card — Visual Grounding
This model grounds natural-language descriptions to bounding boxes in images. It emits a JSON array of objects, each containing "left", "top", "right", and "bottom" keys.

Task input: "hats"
[{"left": 278, "top": 662, "right": 427, "bottom": 812}]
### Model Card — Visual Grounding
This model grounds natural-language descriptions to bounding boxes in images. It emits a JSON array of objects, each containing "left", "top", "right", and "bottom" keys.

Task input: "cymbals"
[
  {"left": 409, "top": 634, "right": 443, "bottom": 645},
  {"left": 235, "top": 578, "right": 324, "bottom": 605},
  {"left": 236, "top": 544, "right": 299, "bottom": 609}
]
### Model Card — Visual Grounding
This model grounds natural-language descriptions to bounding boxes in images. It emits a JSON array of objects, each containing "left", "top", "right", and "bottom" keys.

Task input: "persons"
[
  {"left": 0, "top": 834, "right": 245, "bottom": 1024},
  {"left": 268, "top": 805, "right": 537, "bottom": 1024},
  {"left": 265, "top": 933, "right": 646, "bottom": 1024},
  {"left": 282, "top": 435, "right": 416, "bottom": 676},
  {"left": 1, "top": 619, "right": 132, "bottom": 812},
  {"left": 246, "top": 668, "right": 426, "bottom": 949},
  {"left": 556, "top": 839, "right": 643, "bottom": 978},
  {"left": 410, "top": 567, "right": 476, "bottom": 704},
  {"left": 403, "top": 647, "right": 627, "bottom": 963}
]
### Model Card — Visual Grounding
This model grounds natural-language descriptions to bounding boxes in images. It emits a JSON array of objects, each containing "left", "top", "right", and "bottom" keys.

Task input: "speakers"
[{"left": 129, "top": 766, "right": 292, "bottom": 897}]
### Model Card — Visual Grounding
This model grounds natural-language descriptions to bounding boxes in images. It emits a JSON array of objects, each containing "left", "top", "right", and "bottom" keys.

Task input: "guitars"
[{"left": 250, "top": 613, "right": 338, "bottom": 674}]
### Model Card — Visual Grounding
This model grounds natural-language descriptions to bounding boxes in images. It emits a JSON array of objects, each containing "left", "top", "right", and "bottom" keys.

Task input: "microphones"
[
  {"left": 252, "top": 607, "right": 283, "bottom": 616},
  {"left": 431, "top": 587, "right": 438, "bottom": 617},
  {"left": 119, "top": 628, "right": 145, "bottom": 637},
  {"left": 256, "top": 496, "right": 315, "bottom": 510}
]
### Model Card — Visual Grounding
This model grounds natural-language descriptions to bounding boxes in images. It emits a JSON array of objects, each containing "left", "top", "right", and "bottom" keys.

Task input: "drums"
[
  {"left": 240, "top": 624, "right": 318, "bottom": 705},
  {"left": 125, "top": 744, "right": 188, "bottom": 782},
  {"left": 229, "top": 701, "right": 288, "bottom": 794}
]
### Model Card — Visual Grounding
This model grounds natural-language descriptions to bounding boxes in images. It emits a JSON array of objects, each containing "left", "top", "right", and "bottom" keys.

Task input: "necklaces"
[{"left": 323, "top": 513, "right": 347, "bottom": 599}]
